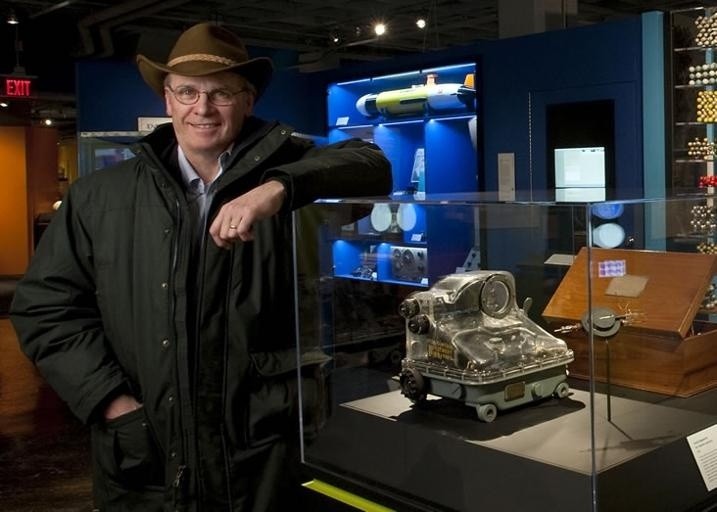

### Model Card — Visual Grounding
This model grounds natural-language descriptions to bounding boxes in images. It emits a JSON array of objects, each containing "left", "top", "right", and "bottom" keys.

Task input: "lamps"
[
  {"left": 44, "top": 117, "right": 55, "bottom": 126},
  {"left": 7, "top": 14, "right": 19, "bottom": 25},
  {"left": 414, "top": 12, "right": 430, "bottom": 29},
  {"left": 0, "top": 98, "right": 11, "bottom": 107}
]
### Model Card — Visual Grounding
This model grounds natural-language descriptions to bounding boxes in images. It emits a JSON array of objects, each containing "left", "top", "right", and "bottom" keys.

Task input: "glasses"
[{"left": 167, "top": 84, "right": 251, "bottom": 106}]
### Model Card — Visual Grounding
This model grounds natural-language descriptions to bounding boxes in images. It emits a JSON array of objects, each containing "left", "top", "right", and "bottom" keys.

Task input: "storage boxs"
[{"left": 541, "top": 246, "right": 717, "bottom": 400}]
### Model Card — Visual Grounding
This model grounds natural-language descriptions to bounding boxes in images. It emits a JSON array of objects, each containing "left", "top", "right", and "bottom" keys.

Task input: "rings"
[{"left": 230, "top": 224, "right": 236, "bottom": 230}]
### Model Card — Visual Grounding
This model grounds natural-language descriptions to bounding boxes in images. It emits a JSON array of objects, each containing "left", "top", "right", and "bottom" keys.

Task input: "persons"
[{"left": 6, "top": 20, "right": 394, "bottom": 511}]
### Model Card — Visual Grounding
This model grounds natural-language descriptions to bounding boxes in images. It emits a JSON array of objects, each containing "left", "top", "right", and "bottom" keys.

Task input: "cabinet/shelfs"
[
  {"left": 325, "top": 62, "right": 479, "bottom": 290},
  {"left": 664, "top": 3, "right": 717, "bottom": 323}
]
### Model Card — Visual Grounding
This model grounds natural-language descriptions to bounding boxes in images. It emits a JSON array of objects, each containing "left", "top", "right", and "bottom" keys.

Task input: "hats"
[{"left": 135, "top": 22, "right": 272, "bottom": 102}]
